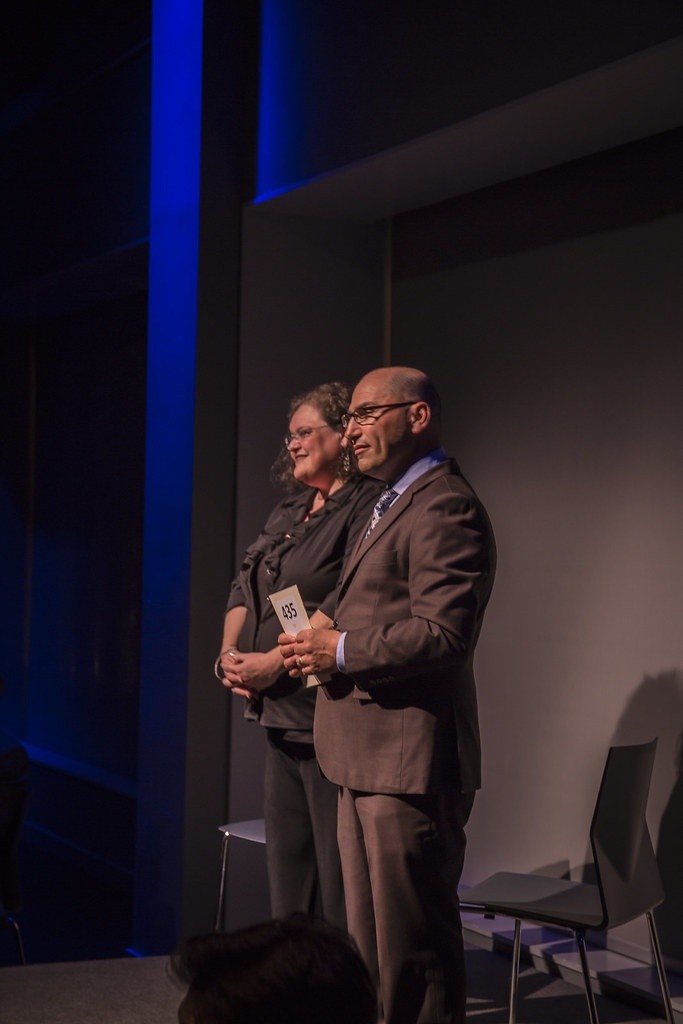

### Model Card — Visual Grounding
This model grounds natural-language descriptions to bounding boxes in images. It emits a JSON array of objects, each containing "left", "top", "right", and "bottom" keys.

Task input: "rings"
[{"left": 299, "top": 656, "right": 303, "bottom": 664}]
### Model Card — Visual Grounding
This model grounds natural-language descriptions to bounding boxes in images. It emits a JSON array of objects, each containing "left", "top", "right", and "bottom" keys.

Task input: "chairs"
[
  {"left": 214, "top": 818, "right": 267, "bottom": 932},
  {"left": 459, "top": 737, "right": 674, "bottom": 1024}
]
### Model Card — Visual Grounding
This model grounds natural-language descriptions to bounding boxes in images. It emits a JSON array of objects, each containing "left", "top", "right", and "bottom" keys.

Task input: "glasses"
[
  {"left": 341, "top": 401, "right": 416, "bottom": 430},
  {"left": 284, "top": 424, "right": 328, "bottom": 447}
]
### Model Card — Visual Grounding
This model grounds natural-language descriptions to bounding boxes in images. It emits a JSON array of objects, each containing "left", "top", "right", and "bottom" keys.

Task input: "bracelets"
[{"left": 214, "top": 648, "right": 237, "bottom": 679}]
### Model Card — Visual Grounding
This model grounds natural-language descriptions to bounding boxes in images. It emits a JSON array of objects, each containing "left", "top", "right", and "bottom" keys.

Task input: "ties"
[{"left": 361, "top": 489, "right": 397, "bottom": 546}]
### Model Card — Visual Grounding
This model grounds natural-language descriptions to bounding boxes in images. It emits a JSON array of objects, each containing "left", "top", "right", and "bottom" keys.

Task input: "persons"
[
  {"left": 213, "top": 381, "right": 387, "bottom": 936},
  {"left": 278, "top": 369, "right": 498, "bottom": 1024},
  {"left": 177, "top": 910, "right": 379, "bottom": 1024}
]
